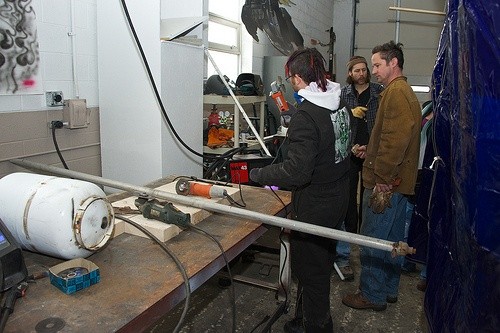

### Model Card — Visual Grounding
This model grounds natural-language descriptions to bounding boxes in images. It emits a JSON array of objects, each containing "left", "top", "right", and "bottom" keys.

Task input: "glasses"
[{"left": 285, "top": 73, "right": 302, "bottom": 83}]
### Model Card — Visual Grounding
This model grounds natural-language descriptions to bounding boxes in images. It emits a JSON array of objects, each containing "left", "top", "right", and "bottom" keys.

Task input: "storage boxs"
[{"left": 49, "top": 258, "right": 100, "bottom": 295}]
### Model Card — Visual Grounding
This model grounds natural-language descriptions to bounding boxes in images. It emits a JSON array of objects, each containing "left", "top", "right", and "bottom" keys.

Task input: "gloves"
[
  {"left": 249, "top": 168, "right": 261, "bottom": 182},
  {"left": 351, "top": 106, "right": 368, "bottom": 119},
  {"left": 367, "top": 184, "right": 392, "bottom": 214}
]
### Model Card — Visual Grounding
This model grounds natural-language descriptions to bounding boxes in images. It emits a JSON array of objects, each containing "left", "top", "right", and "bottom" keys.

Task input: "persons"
[
  {"left": 249, "top": 47, "right": 357, "bottom": 333},
  {"left": 340, "top": 56, "right": 385, "bottom": 234},
  {"left": 404, "top": 100, "right": 434, "bottom": 263},
  {"left": 341, "top": 40, "right": 423, "bottom": 312}
]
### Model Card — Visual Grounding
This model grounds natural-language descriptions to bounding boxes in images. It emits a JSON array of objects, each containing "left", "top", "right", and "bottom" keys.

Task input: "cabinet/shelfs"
[{"left": 203, "top": 94, "right": 266, "bottom": 156}]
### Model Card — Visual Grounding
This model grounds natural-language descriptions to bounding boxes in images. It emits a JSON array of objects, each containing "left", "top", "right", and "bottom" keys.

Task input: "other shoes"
[
  {"left": 338, "top": 263, "right": 355, "bottom": 280},
  {"left": 342, "top": 288, "right": 387, "bottom": 311},
  {"left": 387, "top": 297, "right": 398, "bottom": 304},
  {"left": 283, "top": 318, "right": 298, "bottom": 333},
  {"left": 417, "top": 278, "right": 426, "bottom": 292}
]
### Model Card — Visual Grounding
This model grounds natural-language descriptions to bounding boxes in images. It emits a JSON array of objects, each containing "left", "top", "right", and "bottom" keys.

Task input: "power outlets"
[{"left": 45, "top": 90, "right": 64, "bottom": 107}]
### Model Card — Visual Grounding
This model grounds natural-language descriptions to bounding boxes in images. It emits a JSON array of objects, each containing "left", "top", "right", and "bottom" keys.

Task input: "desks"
[{"left": 0, "top": 174, "right": 291, "bottom": 333}]
